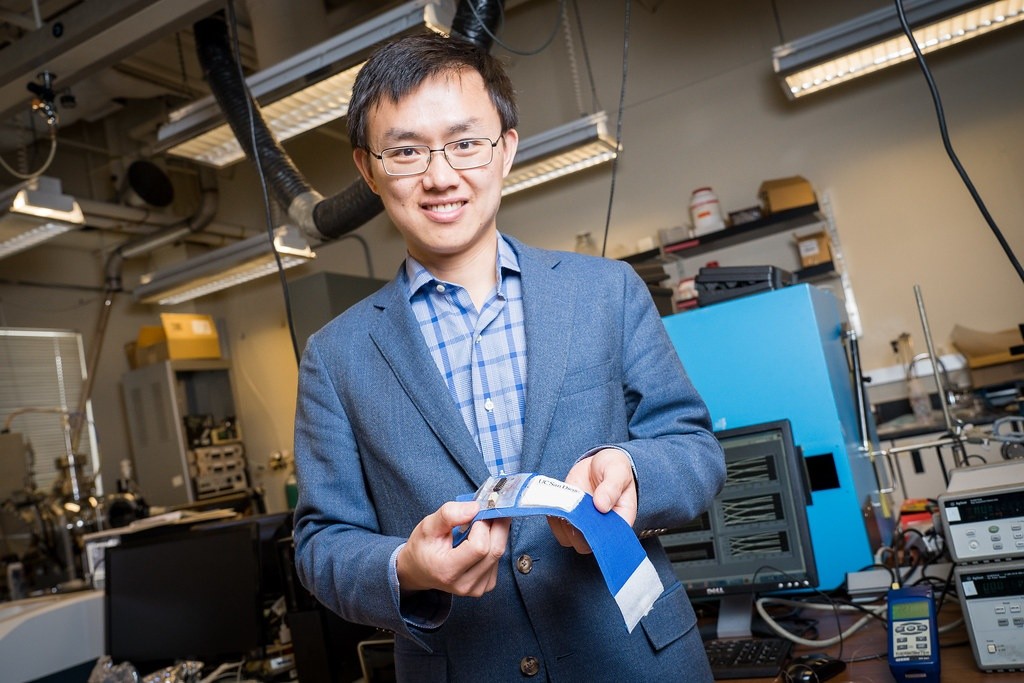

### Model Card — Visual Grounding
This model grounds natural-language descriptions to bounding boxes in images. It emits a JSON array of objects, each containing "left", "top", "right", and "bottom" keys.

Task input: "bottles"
[
  {"left": 575, "top": 230, "right": 599, "bottom": 255},
  {"left": 688, "top": 187, "right": 721, "bottom": 228},
  {"left": 677, "top": 278, "right": 695, "bottom": 296}
]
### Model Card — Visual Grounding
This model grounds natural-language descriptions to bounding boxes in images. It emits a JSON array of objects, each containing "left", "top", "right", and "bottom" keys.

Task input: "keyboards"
[{"left": 704, "top": 638, "right": 794, "bottom": 680}]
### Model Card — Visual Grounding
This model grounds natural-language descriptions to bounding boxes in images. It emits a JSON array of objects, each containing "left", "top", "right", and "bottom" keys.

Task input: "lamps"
[
  {"left": 501, "top": 0, "right": 621, "bottom": 204},
  {"left": 0, "top": 108, "right": 85, "bottom": 259},
  {"left": 133, "top": 229, "right": 310, "bottom": 312},
  {"left": 151, "top": 0, "right": 457, "bottom": 172},
  {"left": 768, "top": 0, "right": 1024, "bottom": 103}
]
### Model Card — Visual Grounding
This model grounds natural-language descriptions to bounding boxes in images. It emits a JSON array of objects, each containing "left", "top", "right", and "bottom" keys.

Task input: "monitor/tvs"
[
  {"left": 656, "top": 418, "right": 819, "bottom": 639},
  {"left": 104, "top": 523, "right": 260, "bottom": 678}
]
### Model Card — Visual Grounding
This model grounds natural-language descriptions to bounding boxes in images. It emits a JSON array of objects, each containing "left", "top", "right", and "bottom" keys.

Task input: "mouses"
[{"left": 780, "top": 652, "right": 847, "bottom": 683}]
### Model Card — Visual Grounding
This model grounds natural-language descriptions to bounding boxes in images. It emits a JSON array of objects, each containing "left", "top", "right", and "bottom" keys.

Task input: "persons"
[{"left": 292, "top": 33, "right": 727, "bottom": 683}]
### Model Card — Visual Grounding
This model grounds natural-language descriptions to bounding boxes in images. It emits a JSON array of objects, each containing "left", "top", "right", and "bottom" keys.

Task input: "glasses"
[{"left": 366, "top": 134, "right": 503, "bottom": 175}]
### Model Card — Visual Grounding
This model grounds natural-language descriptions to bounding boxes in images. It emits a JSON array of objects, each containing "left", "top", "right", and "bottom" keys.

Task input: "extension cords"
[{"left": 848, "top": 561, "right": 958, "bottom": 601}]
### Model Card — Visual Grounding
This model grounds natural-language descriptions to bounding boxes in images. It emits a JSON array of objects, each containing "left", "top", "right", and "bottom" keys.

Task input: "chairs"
[{"left": 103, "top": 505, "right": 297, "bottom": 683}]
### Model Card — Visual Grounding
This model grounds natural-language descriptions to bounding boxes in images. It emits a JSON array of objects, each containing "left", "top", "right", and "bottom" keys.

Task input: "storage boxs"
[
  {"left": 759, "top": 176, "right": 835, "bottom": 269},
  {"left": 128, "top": 313, "right": 216, "bottom": 367}
]
